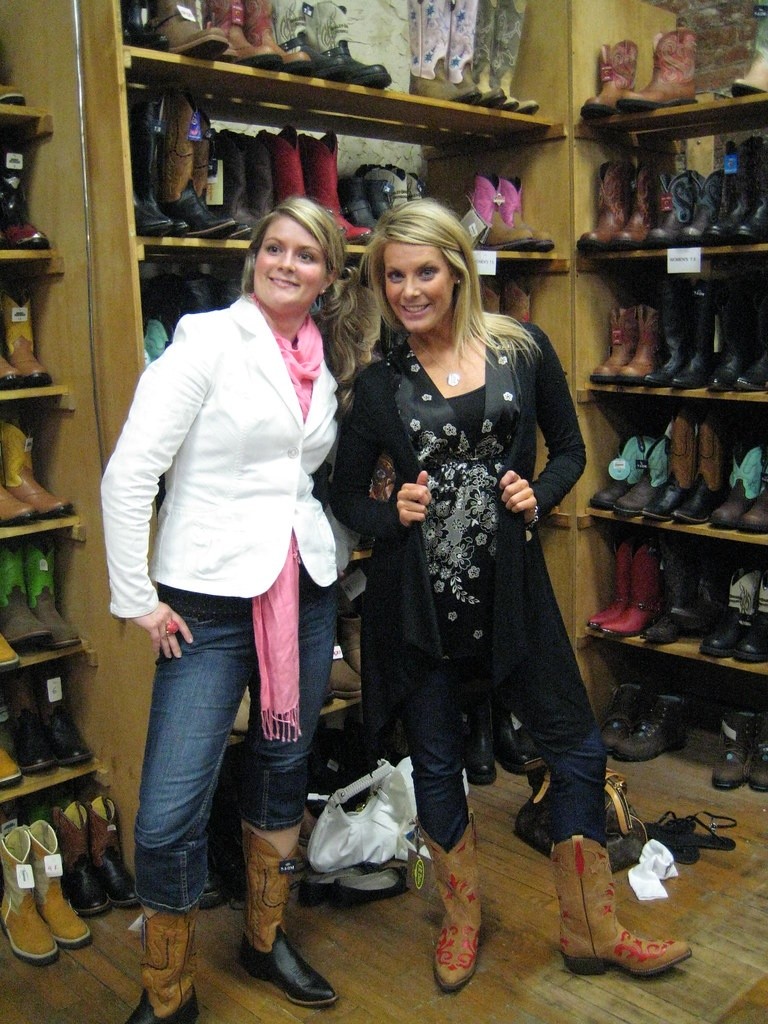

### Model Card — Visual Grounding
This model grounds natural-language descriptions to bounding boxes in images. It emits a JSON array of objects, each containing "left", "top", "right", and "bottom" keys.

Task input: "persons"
[
  {"left": 327, "top": 199, "right": 693, "bottom": 994},
  {"left": 100, "top": 194, "right": 362, "bottom": 1024}
]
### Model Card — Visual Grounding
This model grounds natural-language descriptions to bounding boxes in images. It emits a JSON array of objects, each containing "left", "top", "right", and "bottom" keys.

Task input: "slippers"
[{"left": 645, "top": 810, "right": 737, "bottom": 866}]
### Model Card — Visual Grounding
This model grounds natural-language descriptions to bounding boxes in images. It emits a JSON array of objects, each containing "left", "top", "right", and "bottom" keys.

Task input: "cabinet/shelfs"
[
  {"left": 122, "top": 39, "right": 573, "bottom": 753},
  {"left": 0, "top": 103, "right": 97, "bottom": 805},
  {"left": 578, "top": 89, "right": 768, "bottom": 725}
]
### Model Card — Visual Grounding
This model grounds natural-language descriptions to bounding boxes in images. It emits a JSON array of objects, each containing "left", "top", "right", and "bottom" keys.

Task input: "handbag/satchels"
[
  {"left": 305, "top": 759, "right": 419, "bottom": 872},
  {"left": 514, "top": 754, "right": 643, "bottom": 874}
]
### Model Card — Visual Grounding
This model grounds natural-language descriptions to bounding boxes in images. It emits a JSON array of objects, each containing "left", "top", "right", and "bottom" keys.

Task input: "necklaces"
[{"left": 411, "top": 335, "right": 462, "bottom": 387}]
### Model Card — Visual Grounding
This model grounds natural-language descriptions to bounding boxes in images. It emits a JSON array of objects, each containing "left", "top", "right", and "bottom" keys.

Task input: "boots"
[
  {"left": 235, "top": 825, "right": 339, "bottom": 1008},
  {"left": 551, "top": 833, "right": 692, "bottom": 975},
  {"left": 414, "top": 809, "right": 483, "bottom": 990},
  {"left": 0, "top": 794, "right": 139, "bottom": 963},
  {"left": 122, "top": 892, "right": 202, "bottom": 1024},
  {"left": 0, "top": 0, "right": 768, "bottom": 797}
]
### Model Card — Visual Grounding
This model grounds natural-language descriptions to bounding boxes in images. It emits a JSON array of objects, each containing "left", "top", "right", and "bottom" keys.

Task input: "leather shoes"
[
  {"left": 329, "top": 864, "right": 408, "bottom": 908},
  {"left": 297, "top": 861, "right": 380, "bottom": 907}
]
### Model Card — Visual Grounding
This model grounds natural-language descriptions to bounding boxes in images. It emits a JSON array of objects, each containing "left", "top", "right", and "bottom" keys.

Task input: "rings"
[{"left": 166, "top": 620, "right": 179, "bottom": 634}]
